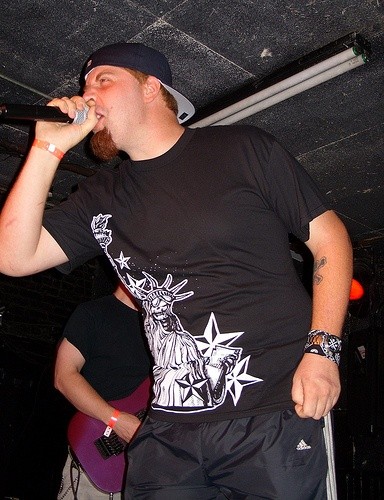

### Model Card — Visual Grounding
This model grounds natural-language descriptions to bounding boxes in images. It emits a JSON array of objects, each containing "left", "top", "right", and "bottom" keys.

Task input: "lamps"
[
  {"left": 348, "top": 257, "right": 376, "bottom": 320},
  {"left": 181, "top": 31, "right": 372, "bottom": 129}
]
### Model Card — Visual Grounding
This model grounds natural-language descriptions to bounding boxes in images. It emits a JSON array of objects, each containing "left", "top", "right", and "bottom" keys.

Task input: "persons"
[
  {"left": 108, "top": 409, "right": 121, "bottom": 427},
  {"left": 54, "top": 281, "right": 155, "bottom": 500},
  {"left": 0, "top": 42, "right": 353, "bottom": 500}
]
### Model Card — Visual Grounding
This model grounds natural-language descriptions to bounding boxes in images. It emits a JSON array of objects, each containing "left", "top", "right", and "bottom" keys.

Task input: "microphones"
[{"left": 0, "top": 103, "right": 90, "bottom": 125}]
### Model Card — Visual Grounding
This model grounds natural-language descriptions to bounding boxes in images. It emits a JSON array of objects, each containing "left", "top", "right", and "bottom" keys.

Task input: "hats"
[{"left": 84, "top": 42, "right": 196, "bottom": 124}]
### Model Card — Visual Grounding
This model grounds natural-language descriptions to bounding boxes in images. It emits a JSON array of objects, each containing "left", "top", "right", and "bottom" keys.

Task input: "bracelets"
[
  {"left": 303, "top": 329, "right": 342, "bottom": 364},
  {"left": 32, "top": 139, "right": 65, "bottom": 160}
]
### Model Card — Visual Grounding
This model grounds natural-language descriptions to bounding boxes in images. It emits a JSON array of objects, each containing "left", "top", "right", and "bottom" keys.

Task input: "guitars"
[{"left": 67, "top": 374, "right": 155, "bottom": 494}]
